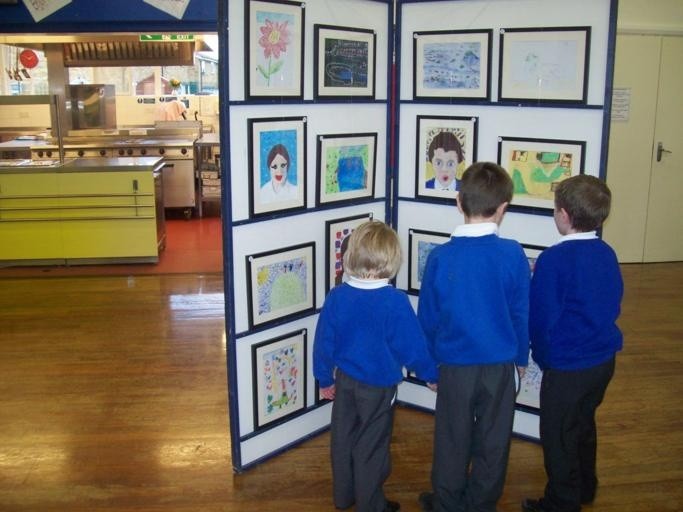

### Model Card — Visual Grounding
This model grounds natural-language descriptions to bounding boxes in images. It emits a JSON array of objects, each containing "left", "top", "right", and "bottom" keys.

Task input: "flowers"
[{"left": 170, "top": 78, "right": 181, "bottom": 89}]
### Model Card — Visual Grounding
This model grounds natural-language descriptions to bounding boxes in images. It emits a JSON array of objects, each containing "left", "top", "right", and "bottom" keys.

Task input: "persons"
[
  {"left": 416, "top": 161, "right": 531, "bottom": 512},
  {"left": 312, "top": 220, "right": 439, "bottom": 512},
  {"left": 260, "top": 144, "right": 297, "bottom": 206},
  {"left": 425, "top": 132, "right": 464, "bottom": 191},
  {"left": 521, "top": 173, "right": 625, "bottom": 511}
]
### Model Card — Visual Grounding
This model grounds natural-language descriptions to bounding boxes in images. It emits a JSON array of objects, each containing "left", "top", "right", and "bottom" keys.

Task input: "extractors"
[{"left": 62, "top": 41, "right": 196, "bottom": 67}]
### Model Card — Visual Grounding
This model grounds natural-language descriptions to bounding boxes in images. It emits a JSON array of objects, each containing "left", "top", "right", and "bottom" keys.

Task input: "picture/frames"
[
  {"left": 498, "top": 135, "right": 586, "bottom": 217},
  {"left": 414, "top": 113, "right": 479, "bottom": 205},
  {"left": 315, "top": 132, "right": 378, "bottom": 208},
  {"left": 403, "top": 370, "right": 438, "bottom": 389},
  {"left": 494, "top": 24, "right": 592, "bottom": 105},
  {"left": 250, "top": 330, "right": 308, "bottom": 429},
  {"left": 243, "top": 0, "right": 306, "bottom": 103},
  {"left": 519, "top": 243, "right": 548, "bottom": 282},
  {"left": 407, "top": 228, "right": 452, "bottom": 291},
  {"left": 326, "top": 212, "right": 375, "bottom": 297},
  {"left": 512, "top": 342, "right": 544, "bottom": 414},
  {"left": 312, "top": 23, "right": 378, "bottom": 104},
  {"left": 411, "top": 28, "right": 495, "bottom": 104},
  {"left": 315, "top": 365, "right": 338, "bottom": 406},
  {"left": 243, "top": 241, "right": 317, "bottom": 329},
  {"left": 245, "top": 115, "right": 308, "bottom": 221}
]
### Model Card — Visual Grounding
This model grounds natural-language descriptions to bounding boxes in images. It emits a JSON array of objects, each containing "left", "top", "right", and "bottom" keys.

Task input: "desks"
[{"left": 194, "top": 132, "right": 221, "bottom": 218}]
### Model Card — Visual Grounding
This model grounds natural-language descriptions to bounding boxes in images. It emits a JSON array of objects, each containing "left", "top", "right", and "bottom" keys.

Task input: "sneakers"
[
  {"left": 419, "top": 492, "right": 438, "bottom": 512},
  {"left": 380, "top": 500, "right": 400, "bottom": 511},
  {"left": 522, "top": 498, "right": 581, "bottom": 511}
]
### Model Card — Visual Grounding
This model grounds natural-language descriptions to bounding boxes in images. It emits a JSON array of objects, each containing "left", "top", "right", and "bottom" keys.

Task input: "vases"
[{"left": 171, "top": 89, "right": 177, "bottom": 95}]
